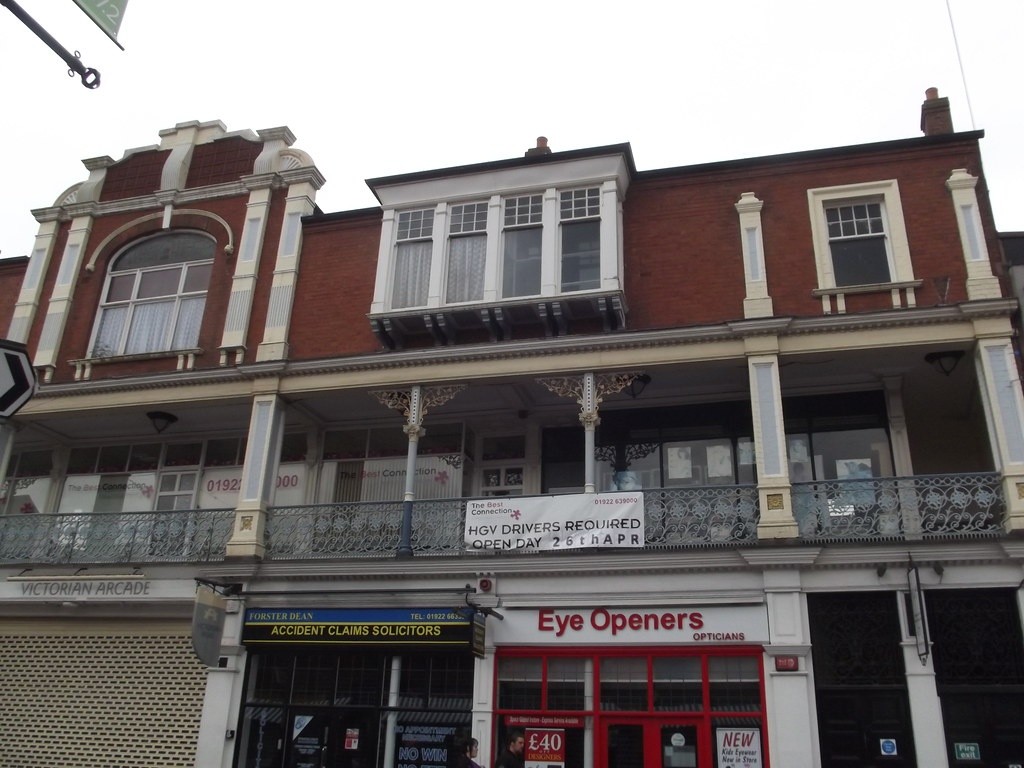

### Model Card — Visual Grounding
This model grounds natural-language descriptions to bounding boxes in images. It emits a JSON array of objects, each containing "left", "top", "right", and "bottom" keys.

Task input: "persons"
[
  {"left": 496, "top": 731, "right": 526, "bottom": 768},
  {"left": 452, "top": 738, "right": 486, "bottom": 768}
]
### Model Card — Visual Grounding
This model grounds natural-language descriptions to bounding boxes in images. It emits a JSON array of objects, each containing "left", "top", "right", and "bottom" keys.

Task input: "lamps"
[
  {"left": 924, "top": 352, "right": 964, "bottom": 374},
  {"left": 616, "top": 373, "right": 650, "bottom": 399},
  {"left": 147, "top": 411, "right": 178, "bottom": 434}
]
